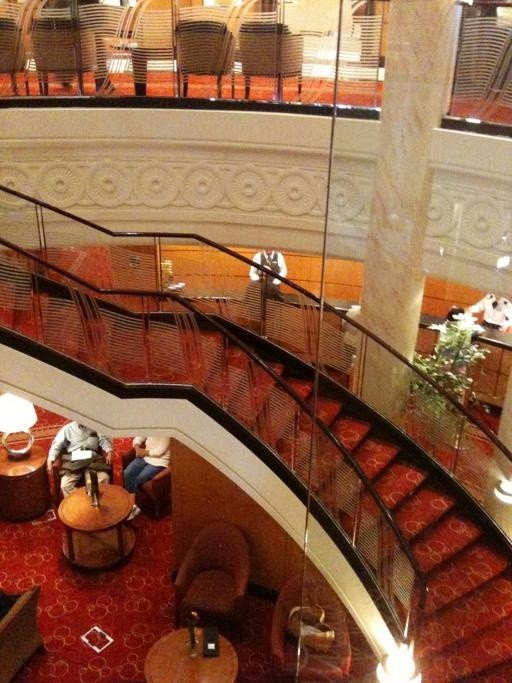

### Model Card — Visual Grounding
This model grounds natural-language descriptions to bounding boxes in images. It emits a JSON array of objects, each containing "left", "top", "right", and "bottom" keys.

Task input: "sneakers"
[{"left": 127, "top": 504, "right": 140, "bottom": 520}]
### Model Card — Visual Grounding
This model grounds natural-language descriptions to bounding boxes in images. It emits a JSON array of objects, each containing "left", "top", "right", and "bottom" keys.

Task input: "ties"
[
  {"left": 268, "top": 255, "right": 272, "bottom": 265},
  {"left": 493, "top": 301, "right": 497, "bottom": 309}
]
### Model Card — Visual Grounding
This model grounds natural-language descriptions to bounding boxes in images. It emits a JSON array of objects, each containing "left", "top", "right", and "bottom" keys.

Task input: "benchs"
[{"left": 271, "top": 574, "right": 352, "bottom": 683}]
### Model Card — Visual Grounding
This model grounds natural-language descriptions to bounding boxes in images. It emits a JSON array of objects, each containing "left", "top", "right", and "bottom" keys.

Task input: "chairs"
[
  {"left": 0, "top": 585, "right": 46, "bottom": 683},
  {"left": 175, "top": 523, "right": 251, "bottom": 634},
  {"left": 123, "top": 444, "right": 172, "bottom": 517},
  {"left": 52, "top": 444, "right": 113, "bottom": 502}
]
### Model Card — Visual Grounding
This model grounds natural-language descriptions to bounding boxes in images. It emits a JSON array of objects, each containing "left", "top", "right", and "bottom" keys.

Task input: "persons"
[
  {"left": 243, "top": 264, "right": 285, "bottom": 332},
  {"left": 124, "top": 436, "right": 171, "bottom": 521},
  {"left": 43, "top": 0, "right": 117, "bottom": 94},
  {"left": 340, "top": 296, "right": 364, "bottom": 389},
  {"left": 436, "top": 305, "right": 486, "bottom": 414},
  {"left": 248, "top": 248, "right": 288, "bottom": 287},
  {"left": 46, "top": 421, "right": 115, "bottom": 498},
  {"left": 471, "top": 292, "right": 511, "bottom": 335}
]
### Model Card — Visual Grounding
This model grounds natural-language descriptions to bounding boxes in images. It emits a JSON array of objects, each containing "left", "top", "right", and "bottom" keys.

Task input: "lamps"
[{"left": 0, "top": 394, "right": 38, "bottom": 460}]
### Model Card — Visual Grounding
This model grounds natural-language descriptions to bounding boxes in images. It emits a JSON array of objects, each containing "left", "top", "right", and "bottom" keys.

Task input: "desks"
[{"left": 0, "top": 445, "right": 47, "bottom": 522}]
